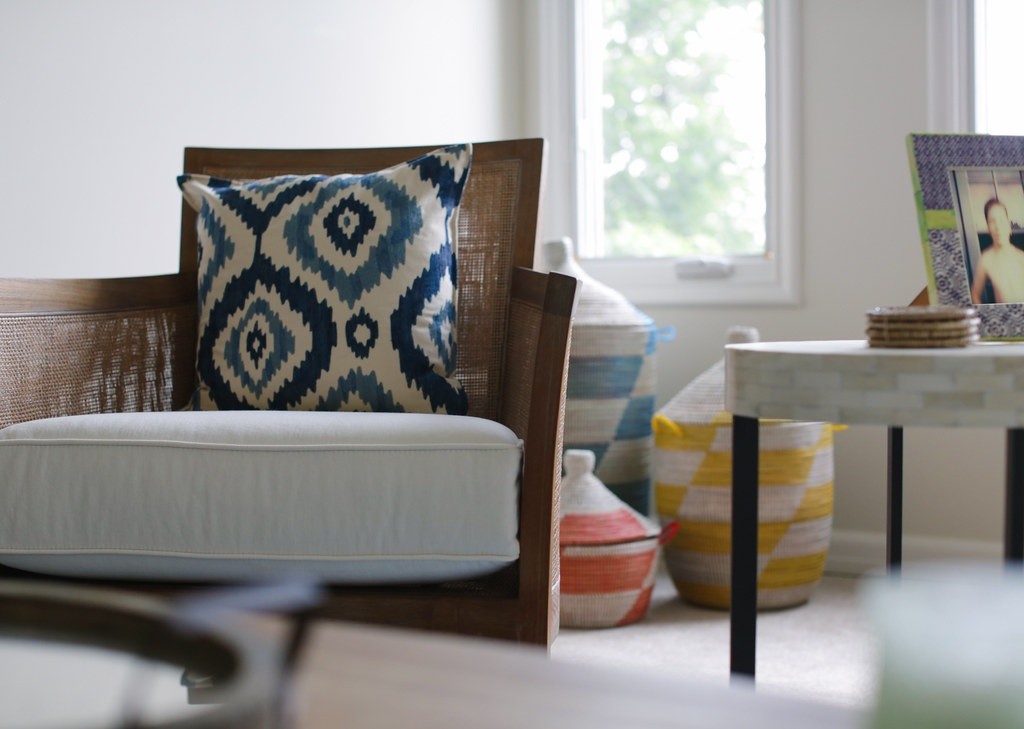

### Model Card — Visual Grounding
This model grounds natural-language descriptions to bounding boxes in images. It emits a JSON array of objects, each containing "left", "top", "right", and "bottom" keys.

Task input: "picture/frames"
[{"left": 903, "top": 131, "right": 1024, "bottom": 345}]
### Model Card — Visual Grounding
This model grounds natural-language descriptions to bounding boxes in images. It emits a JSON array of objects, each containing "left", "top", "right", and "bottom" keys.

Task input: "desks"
[{"left": 724, "top": 337, "right": 1024, "bottom": 679}]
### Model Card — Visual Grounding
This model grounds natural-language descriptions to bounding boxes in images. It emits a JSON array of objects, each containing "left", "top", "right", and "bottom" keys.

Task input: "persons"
[{"left": 972, "top": 200, "right": 1024, "bottom": 305}]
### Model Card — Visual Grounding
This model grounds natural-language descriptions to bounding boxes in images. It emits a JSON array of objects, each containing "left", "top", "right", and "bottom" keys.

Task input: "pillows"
[{"left": 177, "top": 142, "right": 471, "bottom": 416}]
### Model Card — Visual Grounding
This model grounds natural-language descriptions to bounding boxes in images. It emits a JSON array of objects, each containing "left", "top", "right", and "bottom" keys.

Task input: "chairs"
[{"left": 0, "top": 135, "right": 581, "bottom": 646}]
